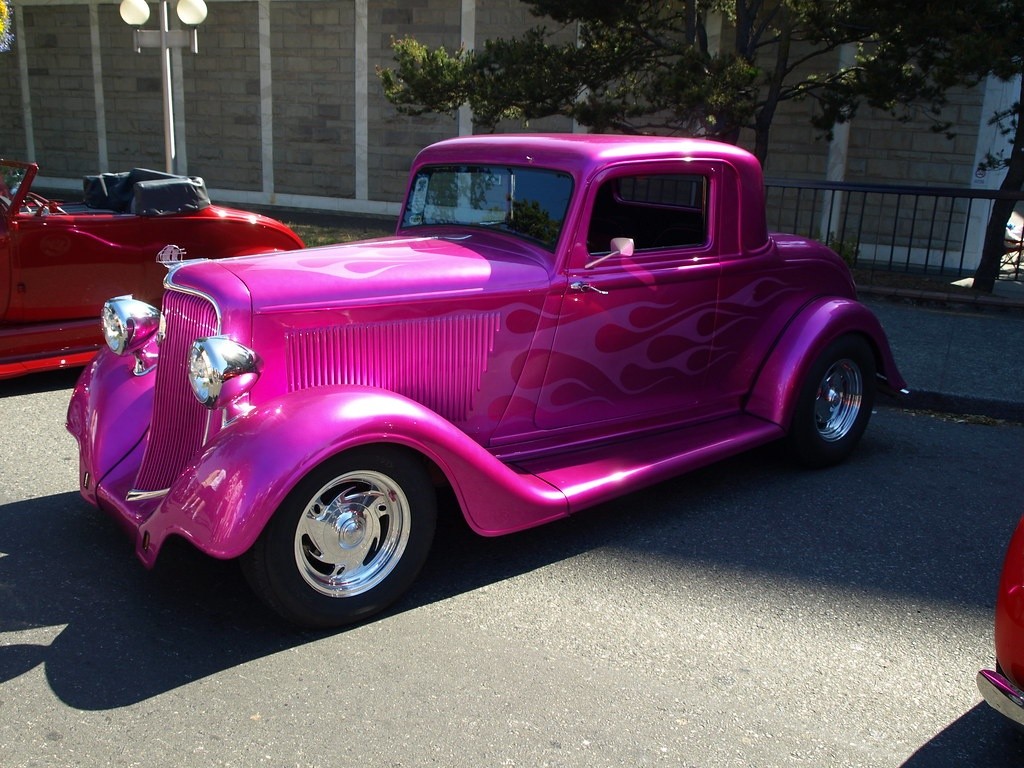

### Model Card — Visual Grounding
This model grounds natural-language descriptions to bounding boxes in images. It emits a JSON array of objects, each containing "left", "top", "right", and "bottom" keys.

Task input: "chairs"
[
  {"left": 652, "top": 226, "right": 703, "bottom": 248},
  {"left": 590, "top": 217, "right": 624, "bottom": 252}
]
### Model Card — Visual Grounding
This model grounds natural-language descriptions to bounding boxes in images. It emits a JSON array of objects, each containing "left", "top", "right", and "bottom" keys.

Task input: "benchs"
[{"left": 81, "top": 168, "right": 211, "bottom": 215}]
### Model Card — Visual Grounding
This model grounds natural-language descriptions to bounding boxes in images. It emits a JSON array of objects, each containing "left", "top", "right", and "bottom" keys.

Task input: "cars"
[
  {"left": 64, "top": 134, "right": 907, "bottom": 626},
  {"left": 978, "top": 514, "right": 1024, "bottom": 727},
  {"left": 0, "top": 157, "right": 305, "bottom": 380}
]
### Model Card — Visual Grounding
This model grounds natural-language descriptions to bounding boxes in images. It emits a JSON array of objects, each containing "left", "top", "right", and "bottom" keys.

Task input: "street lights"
[{"left": 120, "top": 0, "right": 208, "bottom": 175}]
